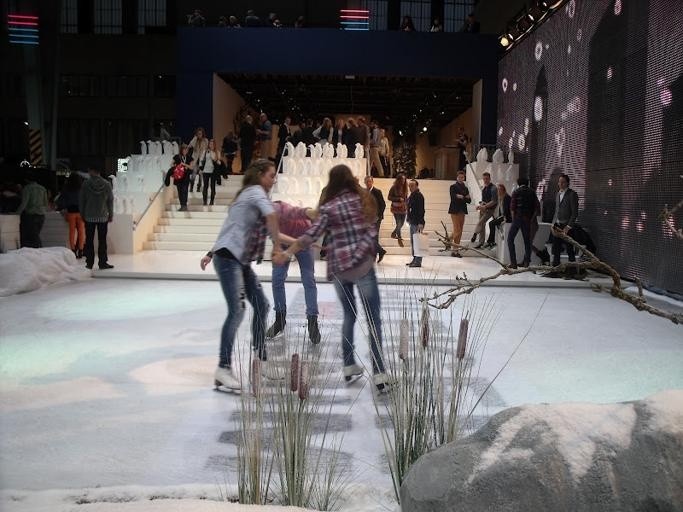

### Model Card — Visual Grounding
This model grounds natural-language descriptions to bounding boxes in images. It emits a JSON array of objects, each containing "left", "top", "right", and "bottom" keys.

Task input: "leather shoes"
[
  {"left": 473, "top": 244, "right": 482, "bottom": 249},
  {"left": 471, "top": 234, "right": 476, "bottom": 242},
  {"left": 484, "top": 243, "right": 493, "bottom": 247},
  {"left": 518, "top": 264, "right": 530, "bottom": 268},
  {"left": 377, "top": 250, "right": 387, "bottom": 264},
  {"left": 451, "top": 252, "right": 461, "bottom": 258},
  {"left": 446, "top": 239, "right": 451, "bottom": 250},
  {"left": 507, "top": 264, "right": 516, "bottom": 269},
  {"left": 406, "top": 261, "right": 413, "bottom": 265},
  {"left": 409, "top": 263, "right": 421, "bottom": 267},
  {"left": 85, "top": 265, "right": 92, "bottom": 269},
  {"left": 98, "top": 263, "right": 113, "bottom": 269}
]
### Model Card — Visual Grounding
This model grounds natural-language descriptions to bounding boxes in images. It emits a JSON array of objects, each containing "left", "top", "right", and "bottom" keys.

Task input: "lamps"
[
  {"left": 499, "top": 32, "right": 516, "bottom": 47},
  {"left": 514, "top": 12, "right": 535, "bottom": 35}
]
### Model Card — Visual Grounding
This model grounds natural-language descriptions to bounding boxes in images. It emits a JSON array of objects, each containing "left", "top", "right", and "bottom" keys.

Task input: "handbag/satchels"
[
  {"left": 413, "top": 228, "right": 430, "bottom": 258},
  {"left": 173, "top": 164, "right": 184, "bottom": 179},
  {"left": 392, "top": 201, "right": 402, "bottom": 207},
  {"left": 198, "top": 152, "right": 207, "bottom": 170}
]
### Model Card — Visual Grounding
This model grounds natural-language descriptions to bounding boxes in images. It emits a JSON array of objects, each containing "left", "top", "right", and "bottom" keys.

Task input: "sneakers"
[
  {"left": 391, "top": 233, "right": 397, "bottom": 238},
  {"left": 320, "top": 255, "right": 328, "bottom": 260},
  {"left": 255, "top": 361, "right": 284, "bottom": 379},
  {"left": 214, "top": 368, "right": 244, "bottom": 391},
  {"left": 373, "top": 373, "right": 399, "bottom": 390},
  {"left": 266, "top": 319, "right": 286, "bottom": 337},
  {"left": 398, "top": 240, "right": 404, "bottom": 247},
  {"left": 344, "top": 364, "right": 363, "bottom": 381},
  {"left": 307, "top": 319, "right": 321, "bottom": 344}
]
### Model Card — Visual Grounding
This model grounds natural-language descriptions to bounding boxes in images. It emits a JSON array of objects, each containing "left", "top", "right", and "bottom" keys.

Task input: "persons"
[
  {"left": 186, "top": 7, "right": 281, "bottom": 29},
  {"left": 171, "top": 143, "right": 194, "bottom": 211},
  {"left": 16, "top": 170, "right": 48, "bottom": 250},
  {"left": 266, "top": 198, "right": 324, "bottom": 346},
  {"left": 470, "top": 172, "right": 498, "bottom": 249},
  {"left": 430, "top": 19, "right": 444, "bottom": 32},
  {"left": 199, "top": 140, "right": 221, "bottom": 205},
  {"left": 272, "top": 164, "right": 400, "bottom": 395},
  {"left": 363, "top": 175, "right": 386, "bottom": 263},
  {"left": 551, "top": 174, "right": 579, "bottom": 281},
  {"left": 189, "top": 128, "right": 209, "bottom": 192},
  {"left": 399, "top": 15, "right": 415, "bottom": 32},
  {"left": 58, "top": 172, "right": 86, "bottom": 259},
  {"left": 239, "top": 115, "right": 255, "bottom": 171},
  {"left": 274, "top": 115, "right": 390, "bottom": 175},
  {"left": 459, "top": 13, "right": 481, "bottom": 33},
  {"left": 486, "top": 185, "right": 512, "bottom": 248},
  {"left": 455, "top": 126, "right": 468, "bottom": 170},
  {"left": 221, "top": 129, "right": 238, "bottom": 174},
  {"left": 256, "top": 113, "right": 272, "bottom": 159},
  {"left": 200, "top": 157, "right": 287, "bottom": 398},
  {"left": 506, "top": 177, "right": 541, "bottom": 270},
  {"left": 388, "top": 173, "right": 408, "bottom": 248},
  {"left": 447, "top": 171, "right": 472, "bottom": 259},
  {"left": 79, "top": 166, "right": 115, "bottom": 271},
  {"left": 406, "top": 180, "right": 426, "bottom": 267}
]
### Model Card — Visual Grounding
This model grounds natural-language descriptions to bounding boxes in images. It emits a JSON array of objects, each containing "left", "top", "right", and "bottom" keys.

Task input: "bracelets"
[
  {"left": 282, "top": 250, "right": 290, "bottom": 259},
  {"left": 206, "top": 251, "right": 213, "bottom": 258}
]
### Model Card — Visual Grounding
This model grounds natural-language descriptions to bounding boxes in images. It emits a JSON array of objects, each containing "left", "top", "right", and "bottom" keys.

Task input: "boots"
[
  {"left": 78, "top": 250, "right": 82, "bottom": 258},
  {"left": 72, "top": 250, "right": 76, "bottom": 255}
]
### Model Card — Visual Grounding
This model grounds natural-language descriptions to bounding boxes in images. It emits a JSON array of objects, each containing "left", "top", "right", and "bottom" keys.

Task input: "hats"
[{"left": 84, "top": 157, "right": 101, "bottom": 171}]
